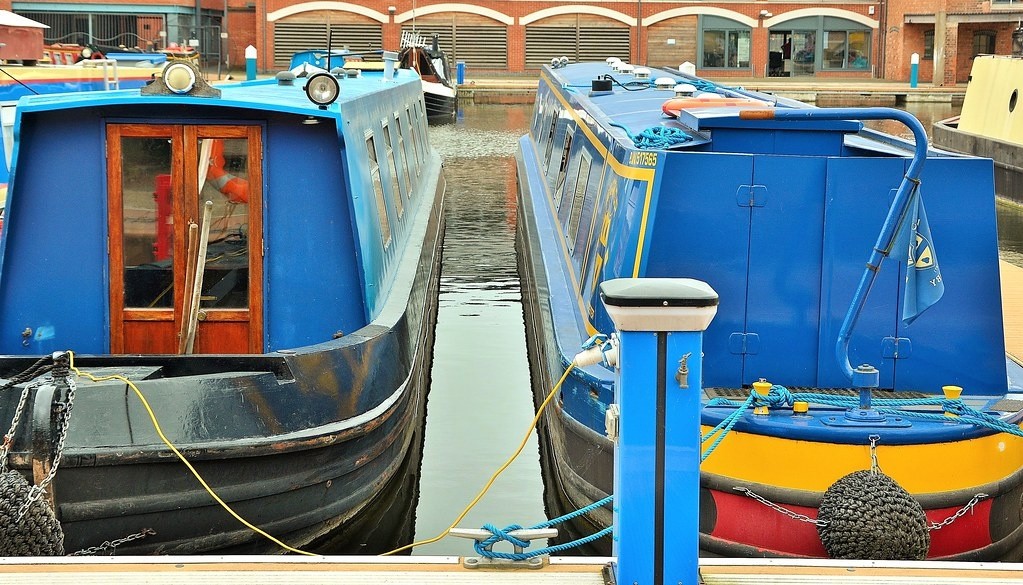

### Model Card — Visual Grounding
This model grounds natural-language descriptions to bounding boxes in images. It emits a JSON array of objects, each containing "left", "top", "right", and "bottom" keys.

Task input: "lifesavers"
[
  {"left": 662, "top": 97, "right": 774, "bottom": 118},
  {"left": 206, "top": 138, "right": 249, "bottom": 205}
]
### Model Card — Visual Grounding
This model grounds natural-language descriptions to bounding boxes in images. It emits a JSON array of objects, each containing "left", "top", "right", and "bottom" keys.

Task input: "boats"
[
  {"left": 516, "top": 57, "right": 1021, "bottom": 565},
  {"left": 932, "top": 54, "right": 1023, "bottom": 212},
  {"left": 397, "top": 1, "right": 459, "bottom": 117},
  {"left": 0, "top": 51, "right": 448, "bottom": 555},
  {"left": 290, "top": 45, "right": 363, "bottom": 79}
]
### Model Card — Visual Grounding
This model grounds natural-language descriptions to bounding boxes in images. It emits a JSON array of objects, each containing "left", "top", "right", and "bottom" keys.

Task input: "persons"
[{"left": 781, "top": 38, "right": 795, "bottom": 59}]
